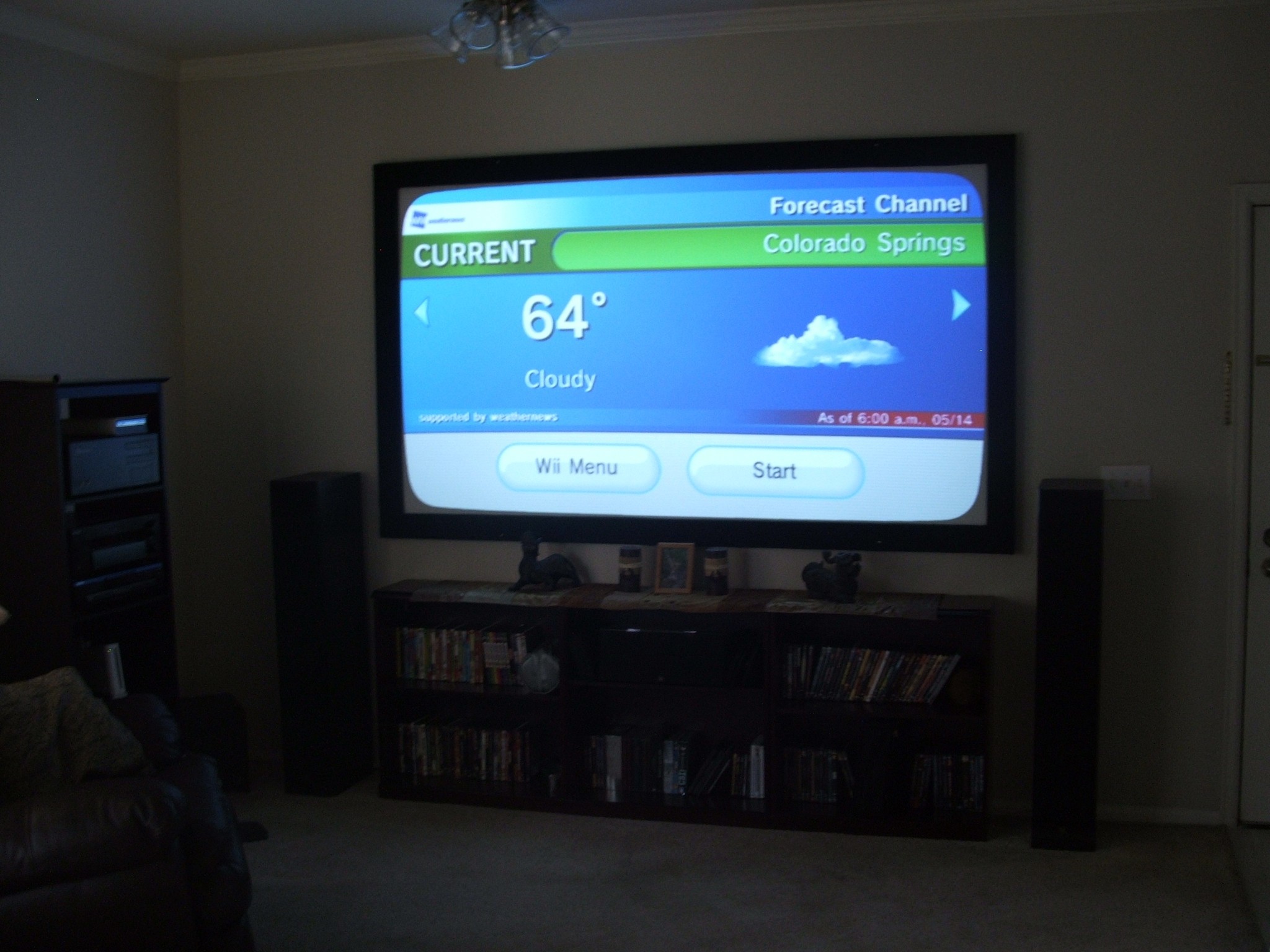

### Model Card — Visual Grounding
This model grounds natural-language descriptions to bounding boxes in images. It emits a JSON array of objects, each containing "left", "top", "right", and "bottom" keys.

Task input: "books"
[
  {"left": 395, "top": 722, "right": 986, "bottom": 810},
  {"left": 787, "top": 642, "right": 962, "bottom": 704},
  {"left": 395, "top": 627, "right": 531, "bottom": 688}
]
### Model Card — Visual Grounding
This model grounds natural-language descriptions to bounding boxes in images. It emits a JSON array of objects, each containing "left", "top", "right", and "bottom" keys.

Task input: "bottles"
[
  {"left": 703, "top": 549, "right": 730, "bottom": 596},
  {"left": 618, "top": 545, "right": 643, "bottom": 592}
]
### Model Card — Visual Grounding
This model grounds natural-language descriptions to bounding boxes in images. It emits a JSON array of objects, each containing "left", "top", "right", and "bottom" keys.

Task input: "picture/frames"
[{"left": 654, "top": 541, "right": 696, "bottom": 594}]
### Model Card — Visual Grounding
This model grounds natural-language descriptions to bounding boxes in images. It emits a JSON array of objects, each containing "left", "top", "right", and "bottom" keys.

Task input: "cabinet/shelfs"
[{"left": 371, "top": 576, "right": 1005, "bottom": 844}]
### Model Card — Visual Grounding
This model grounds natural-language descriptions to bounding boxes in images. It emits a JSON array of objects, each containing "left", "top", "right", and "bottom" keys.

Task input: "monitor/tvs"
[{"left": 373, "top": 133, "right": 1025, "bottom": 557}]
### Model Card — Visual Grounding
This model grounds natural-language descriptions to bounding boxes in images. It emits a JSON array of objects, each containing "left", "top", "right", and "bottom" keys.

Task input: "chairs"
[{"left": 0, "top": 655, "right": 256, "bottom": 952}]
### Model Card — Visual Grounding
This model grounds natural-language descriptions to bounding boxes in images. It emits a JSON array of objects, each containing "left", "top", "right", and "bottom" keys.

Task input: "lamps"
[{"left": 421, "top": 0, "right": 573, "bottom": 73}]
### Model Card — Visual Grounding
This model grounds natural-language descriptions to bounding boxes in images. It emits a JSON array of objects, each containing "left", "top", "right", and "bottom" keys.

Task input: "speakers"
[
  {"left": 1030, "top": 475, "right": 1094, "bottom": 849},
  {"left": 271, "top": 471, "right": 373, "bottom": 795}
]
